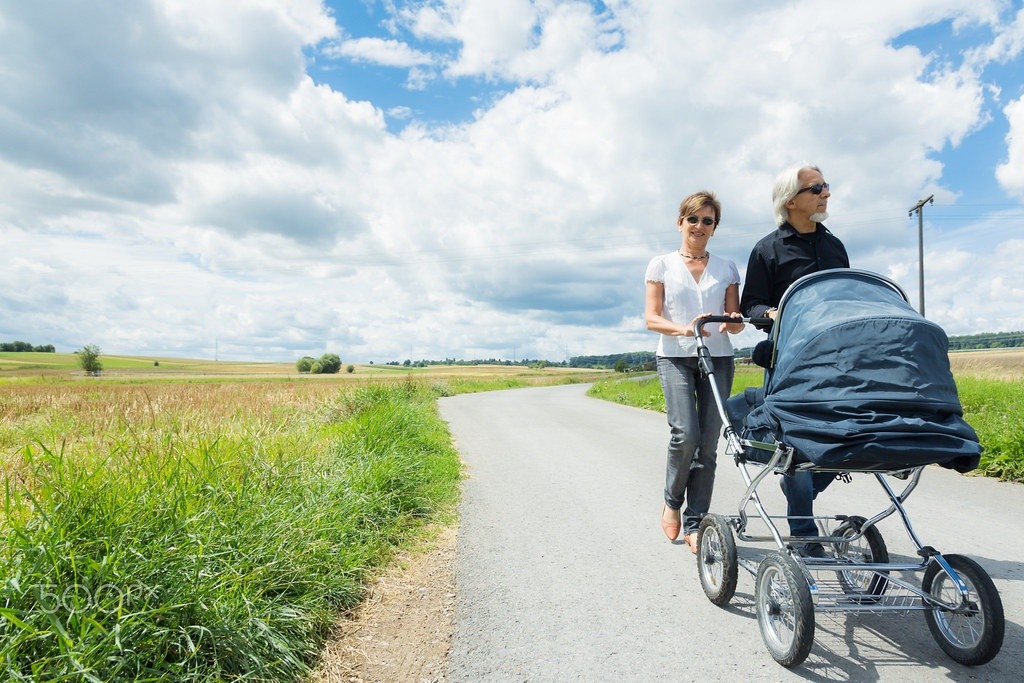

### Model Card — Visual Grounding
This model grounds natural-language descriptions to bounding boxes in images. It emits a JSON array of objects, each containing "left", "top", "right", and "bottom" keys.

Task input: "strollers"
[{"left": 693, "top": 267, "right": 1005, "bottom": 668}]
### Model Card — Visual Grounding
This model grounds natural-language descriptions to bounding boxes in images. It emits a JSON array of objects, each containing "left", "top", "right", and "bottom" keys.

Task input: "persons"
[
  {"left": 643, "top": 190, "right": 746, "bottom": 554},
  {"left": 739, "top": 161, "right": 850, "bottom": 565}
]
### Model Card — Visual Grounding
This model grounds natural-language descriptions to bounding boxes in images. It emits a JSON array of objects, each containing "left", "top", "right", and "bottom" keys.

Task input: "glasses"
[
  {"left": 797, "top": 183, "right": 829, "bottom": 194},
  {"left": 683, "top": 217, "right": 716, "bottom": 225}
]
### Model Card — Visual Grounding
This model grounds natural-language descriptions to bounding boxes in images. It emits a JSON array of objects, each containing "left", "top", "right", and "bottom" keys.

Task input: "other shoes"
[
  {"left": 684, "top": 535, "right": 698, "bottom": 553},
  {"left": 789, "top": 543, "right": 837, "bottom": 564},
  {"left": 779, "top": 477, "right": 790, "bottom": 524},
  {"left": 662, "top": 505, "right": 681, "bottom": 541}
]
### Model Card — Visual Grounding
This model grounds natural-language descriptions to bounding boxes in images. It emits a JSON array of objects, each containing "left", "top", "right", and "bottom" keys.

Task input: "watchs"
[{"left": 766, "top": 307, "right": 778, "bottom": 321}]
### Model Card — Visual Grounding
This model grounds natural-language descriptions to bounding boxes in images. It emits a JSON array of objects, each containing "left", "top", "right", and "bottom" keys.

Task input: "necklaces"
[{"left": 678, "top": 249, "right": 708, "bottom": 260}]
